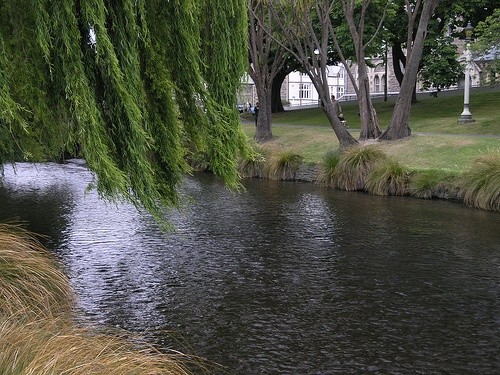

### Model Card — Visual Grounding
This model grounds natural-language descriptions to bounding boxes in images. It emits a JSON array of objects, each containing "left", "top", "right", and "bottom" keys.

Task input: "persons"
[
  {"left": 247, "top": 101, "right": 253, "bottom": 113},
  {"left": 331, "top": 94, "right": 343, "bottom": 116},
  {"left": 338, "top": 111, "right": 350, "bottom": 130},
  {"left": 255, "top": 101, "right": 260, "bottom": 127}
]
